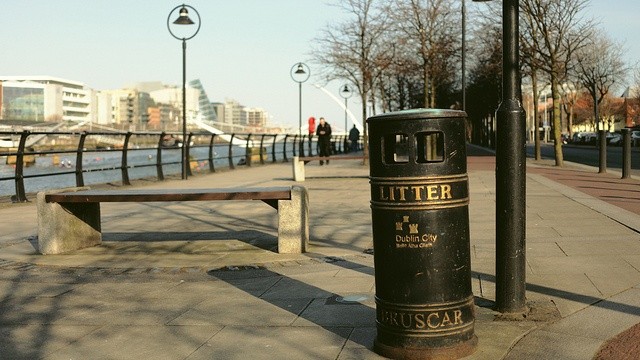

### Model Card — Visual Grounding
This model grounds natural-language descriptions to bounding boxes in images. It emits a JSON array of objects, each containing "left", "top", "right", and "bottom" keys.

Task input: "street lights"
[
  {"left": 291, "top": 62, "right": 310, "bottom": 156},
  {"left": 339, "top": 84, "right": 352, "bottom": 134},
  {"left": 168, "top": 5, "right": 202, "bottom": 178},
  {"left": 366, "top": 97, "right": 373, "bottom": 117}
]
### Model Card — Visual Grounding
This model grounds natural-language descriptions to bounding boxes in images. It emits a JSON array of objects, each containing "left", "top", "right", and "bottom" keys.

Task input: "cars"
[{"left": 609, "top": 131, "right": 640, "bottom": 146}]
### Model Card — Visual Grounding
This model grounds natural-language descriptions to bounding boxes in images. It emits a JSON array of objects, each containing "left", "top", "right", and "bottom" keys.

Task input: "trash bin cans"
[{"left": 366, "top": 108, "right": 477, "bottom": 358}]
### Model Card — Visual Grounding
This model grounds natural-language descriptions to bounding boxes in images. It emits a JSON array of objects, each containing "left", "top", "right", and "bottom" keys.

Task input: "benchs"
[
  {"left": 292, "top": 155, "right": 371, "bottom": 182},
  {"left": 34, "top": 185, "right": 309, "bottom": 254}
]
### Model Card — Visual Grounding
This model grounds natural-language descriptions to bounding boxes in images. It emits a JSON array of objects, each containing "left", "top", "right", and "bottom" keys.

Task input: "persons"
[
  {"left": 316, "top": 117, "right": 331, "bottom": 165},
  {"left": 348, "top": 124, "right": 360, "bottom": 152}
]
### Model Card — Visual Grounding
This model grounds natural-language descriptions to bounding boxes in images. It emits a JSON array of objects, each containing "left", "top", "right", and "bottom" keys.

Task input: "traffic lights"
[{"left": 309, "top": 117, "right": 315, "bottom": 132}]
